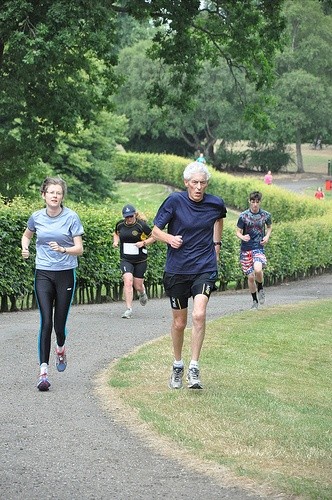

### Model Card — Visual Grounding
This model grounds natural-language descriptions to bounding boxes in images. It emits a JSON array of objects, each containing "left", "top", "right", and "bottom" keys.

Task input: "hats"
[{"left": 122, "top": 205, "right": 135, "bottom": 218}]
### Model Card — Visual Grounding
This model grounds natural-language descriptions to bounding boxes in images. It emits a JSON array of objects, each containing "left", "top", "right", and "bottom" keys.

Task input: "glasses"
[{"left": 126, "top": 216, "right": 133, "bottom": 218}]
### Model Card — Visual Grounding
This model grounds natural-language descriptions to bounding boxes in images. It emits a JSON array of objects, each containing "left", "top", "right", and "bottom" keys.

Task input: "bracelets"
[
  {"left": 213, "top": 242, "right": 222, "bottom": 246},
  {"left": 143, "top": 240, "right": 147, "bottom": 248},
  {"left": 62, "top": 247, "right": 66, "bottom": 253}
]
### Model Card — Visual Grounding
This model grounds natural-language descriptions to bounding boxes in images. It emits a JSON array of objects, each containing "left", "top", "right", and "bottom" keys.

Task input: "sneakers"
[
  {"left": 258, "top": 289, "right": 264, "bottom": 304},
  {"left": 138, "top": 285, "right": 148, "bottom": 306},
  {"left": 170, "top": 362, "right": 185, "bottom": 389},
  {"left": 37, "top": 373, "right": 51, "bottom": 391},
  {"left": 122, "top": 308, "right": 132, "bottom": 318},
  {"left": 55, "top": 344, "right": 67, "bottom": 372},
  {"left": 186, "top": 367, "right": 203, "bottom": 389},
  {"left": 250, "top": 299, "right": 258, "bottom": 310}
]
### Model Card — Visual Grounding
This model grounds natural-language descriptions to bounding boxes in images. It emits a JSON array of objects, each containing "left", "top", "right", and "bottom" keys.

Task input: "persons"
[
  {"left": 112, "top": 204, "right": 157, "bottom": 319},
  {"left": 152, "top": 161, "right": 228, "bottom": 390},
  {"left": 315, "top": 187, "right": 325, "bottom": 201},
  {"left": 236, "top": 191, "right": 273, "bottom": 310},
  {"left": 196, "top": 153, "right": 207, "bottom": 163},
  {"left": 21, "top": 177, "right": 85, "bottom": 391},
  {"left": 264, "top": 171, "right": 273, "bottom": 185}
]
